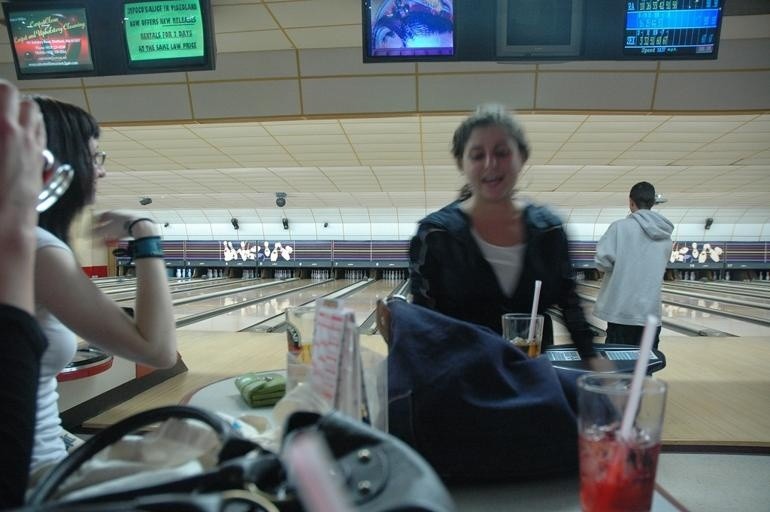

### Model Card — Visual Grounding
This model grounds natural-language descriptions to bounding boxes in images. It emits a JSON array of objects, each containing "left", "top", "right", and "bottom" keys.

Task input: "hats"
[{"left": 630, "top": 182, "right": 655, "bottom": 209}]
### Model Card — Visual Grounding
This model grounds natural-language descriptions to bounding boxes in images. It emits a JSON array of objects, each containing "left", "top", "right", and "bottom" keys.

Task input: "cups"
[
  {"left": 35, "top": 150, "right": 74, "bottom": 215},
  {"left": 573, "top": 368, "right": 670, "bottom": 512},
  {"left": 285, "top": 305, "right": 319, "bottom": 370},
  {"left": 501, "top": 310, "right": 546, "bottom": 361}
]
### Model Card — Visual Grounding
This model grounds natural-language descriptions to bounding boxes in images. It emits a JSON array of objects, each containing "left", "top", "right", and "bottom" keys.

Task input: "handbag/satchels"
[
  {"left": 235, "top": 372, "right": 287, "bottom": 406},
  {"left": 385, "top": 296, "right": 622, "bottom": 490},
  {"left": 26, "top": 406, "right": 459, "bottom": 512}
]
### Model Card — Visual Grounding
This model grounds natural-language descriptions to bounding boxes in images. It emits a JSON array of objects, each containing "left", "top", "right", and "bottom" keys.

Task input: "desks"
[{"left": 154, "top": 366, "right": 693, "bottom": 512}]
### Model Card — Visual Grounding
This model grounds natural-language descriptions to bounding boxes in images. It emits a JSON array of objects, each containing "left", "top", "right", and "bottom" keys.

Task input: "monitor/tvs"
[
  {"left": 361, "top": 0, "right": 459, "bottom": 64},
  {"left": 618, "top": 1, "right": 724, "bottom": 61},
  {"left": 118, "top": 0, "right": 214, "bottom": 71},
  {"left": 495, "top": 0, "right": 582, "bottom": 57},
  {"left": 4, "top": 0, "right": 98, "bottom": 77}
]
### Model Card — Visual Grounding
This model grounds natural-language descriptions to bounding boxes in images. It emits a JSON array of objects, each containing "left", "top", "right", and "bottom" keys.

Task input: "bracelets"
[
  {"left": 130, "top": 218, "right": 154, "bottom": 232},
  {"left": 129, "top": 236, "right": 165, "bottom": 257}
]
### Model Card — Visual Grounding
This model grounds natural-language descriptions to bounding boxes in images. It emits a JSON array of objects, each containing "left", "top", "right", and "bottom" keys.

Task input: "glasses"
[{"left": 89, "top": 151, "right": 107, "bottom": 166}]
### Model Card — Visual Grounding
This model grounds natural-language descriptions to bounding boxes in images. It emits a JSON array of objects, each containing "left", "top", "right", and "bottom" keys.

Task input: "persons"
[
  {"left": 595, "top": 181, "right": 675, "bottom": 377},
  {"left": 1, "top": 81, "right": 49, "bottom": 512},
  {"left": 408, "top": 106, "right": 622, "bottom": 388},
  {"left": 23, "top": 96, "right": 180, "bottom": 511}
]
